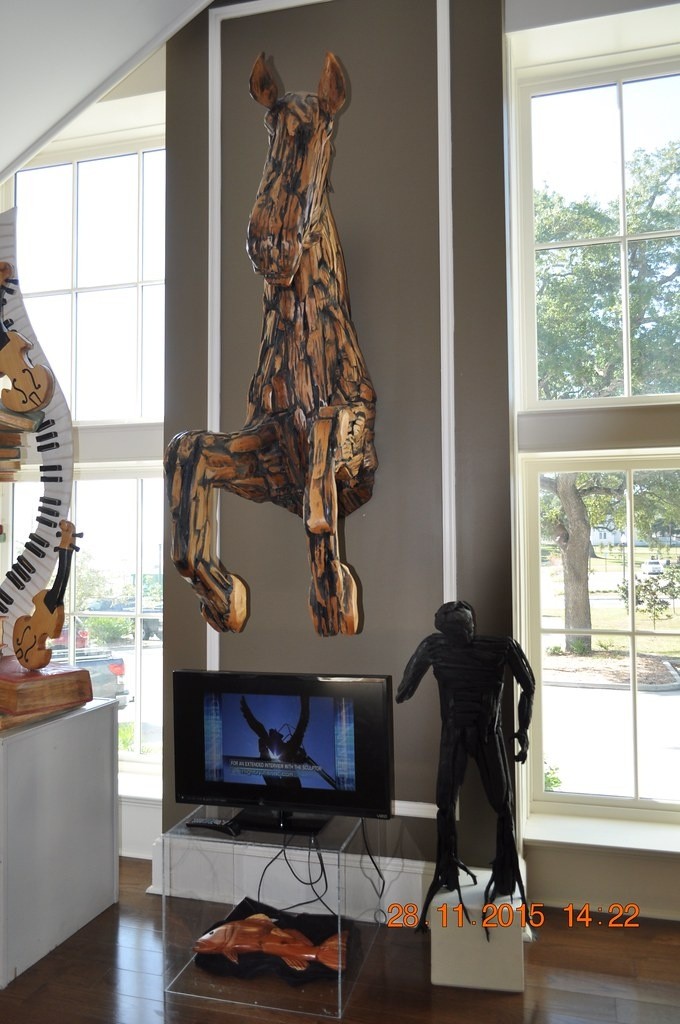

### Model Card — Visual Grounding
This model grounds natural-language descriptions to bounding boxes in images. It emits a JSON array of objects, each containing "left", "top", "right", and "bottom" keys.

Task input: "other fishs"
[{"left": 192, "top": 913, "right": 348, "bottom": 973}]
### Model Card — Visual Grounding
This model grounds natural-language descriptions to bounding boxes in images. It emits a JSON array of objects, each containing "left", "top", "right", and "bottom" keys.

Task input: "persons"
[{"left": 397, "top": 600, "right": 538, "bottom": 898}]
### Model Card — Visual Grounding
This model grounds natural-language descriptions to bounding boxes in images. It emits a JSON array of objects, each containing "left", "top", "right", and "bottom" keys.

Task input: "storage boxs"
[{"left": 158, "top": 809, "right": 381, "bottom": 1019}]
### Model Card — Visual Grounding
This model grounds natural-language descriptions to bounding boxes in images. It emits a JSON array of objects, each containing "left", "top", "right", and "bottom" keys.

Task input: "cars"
[
  {"left": 48, "top": 614, "right": 89, "bottom": 658},
  {"left": 83, "top": 595, "right": 163, "bottom": 641}
]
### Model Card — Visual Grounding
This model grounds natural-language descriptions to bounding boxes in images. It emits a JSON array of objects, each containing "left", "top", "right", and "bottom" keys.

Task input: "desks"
[{"left": 0, "top": 697, "right": 120, "bottom": 991}]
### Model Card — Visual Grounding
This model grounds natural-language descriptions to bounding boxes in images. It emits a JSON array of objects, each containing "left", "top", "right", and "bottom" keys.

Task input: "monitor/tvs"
[{"left": 172, "top": 669, "right": 396, "bottom": 819}]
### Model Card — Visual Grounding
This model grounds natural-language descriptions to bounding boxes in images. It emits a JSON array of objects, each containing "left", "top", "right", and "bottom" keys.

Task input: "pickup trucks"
[{"left": 47, "top": 647, "right": 134, "bottom": 712}]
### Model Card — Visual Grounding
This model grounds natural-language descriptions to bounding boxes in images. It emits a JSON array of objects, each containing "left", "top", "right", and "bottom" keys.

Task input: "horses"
[{"left": 162, "top": 48, "right": 380, "bottom": 643}]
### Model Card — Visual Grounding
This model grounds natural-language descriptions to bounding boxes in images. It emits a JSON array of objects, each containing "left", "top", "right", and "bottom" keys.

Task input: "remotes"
[{"left": 186, "top": 816, "right": 240, "bottom": 836}]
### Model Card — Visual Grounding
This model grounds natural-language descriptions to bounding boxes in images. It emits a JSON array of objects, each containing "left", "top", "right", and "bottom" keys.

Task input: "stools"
[{"left": 432, "top": 868, "right": 525, "bottom": 993}]
